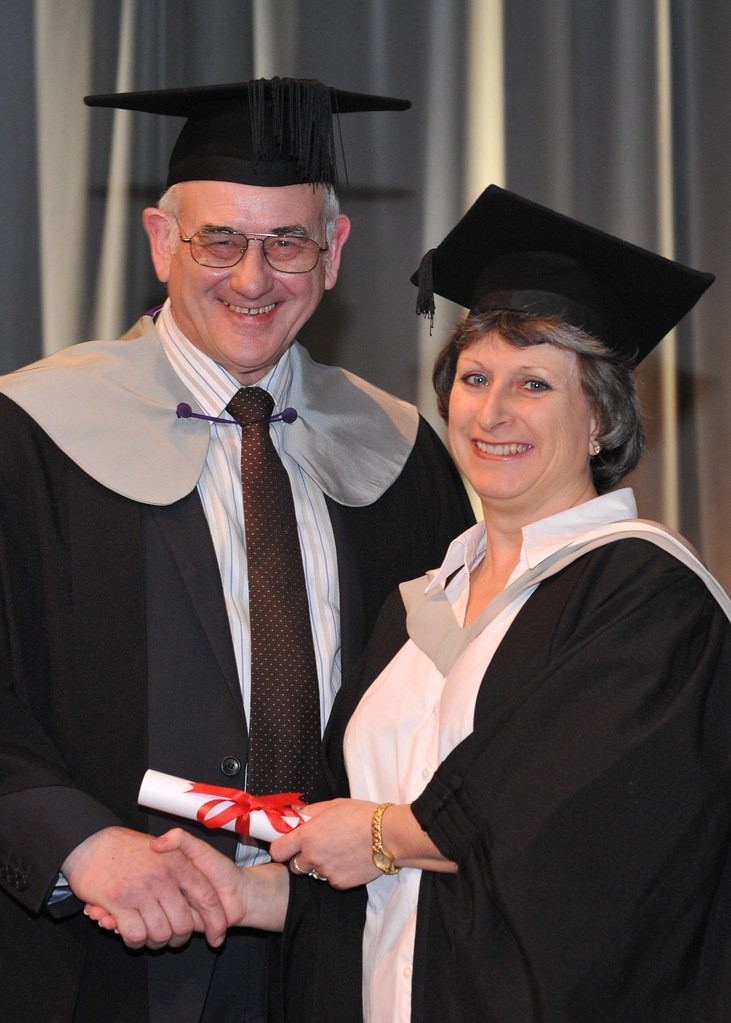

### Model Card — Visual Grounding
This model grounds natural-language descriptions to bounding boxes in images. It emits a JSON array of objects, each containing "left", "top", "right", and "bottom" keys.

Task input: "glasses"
[{"left": 173, "top": 217, "right": 330, "bottom": 275}]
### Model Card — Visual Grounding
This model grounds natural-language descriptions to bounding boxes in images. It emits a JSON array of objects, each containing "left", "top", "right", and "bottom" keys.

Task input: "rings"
[
  {"left": 293, "top": 857, "right": 308, "bottom": 874},
  {"left": 309, "top": 868, "right": 327, "bottom": 881}
]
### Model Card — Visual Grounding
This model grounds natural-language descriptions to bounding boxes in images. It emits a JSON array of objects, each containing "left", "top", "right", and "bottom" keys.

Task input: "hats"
[
  {"left": 83, "top": 78, "right": 413, "bottom": 186},
  {"left": 408, "top": 185, "right": 715, "bottom": 376}
]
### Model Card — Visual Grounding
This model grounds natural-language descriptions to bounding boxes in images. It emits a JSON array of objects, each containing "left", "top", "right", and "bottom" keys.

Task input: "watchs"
[{"left": 371, "top": 803, "right": 401, "bottom": 875}]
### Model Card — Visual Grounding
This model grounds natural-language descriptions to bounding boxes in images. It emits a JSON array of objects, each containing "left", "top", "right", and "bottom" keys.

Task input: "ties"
[{"left": 226, "top": 390, "right": 325, "bottom": 831}]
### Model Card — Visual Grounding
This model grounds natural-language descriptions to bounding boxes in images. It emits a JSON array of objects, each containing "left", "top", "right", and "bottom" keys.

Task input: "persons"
[
  {"left": 0, "top": 77, "right": 478, "bottom": 1023},
  {"left": 88, "top": 184, "right": 731, "bottom": 1023}
]
